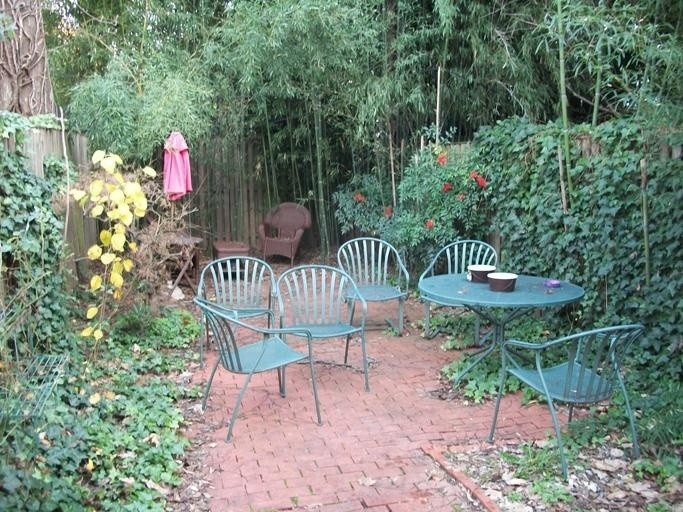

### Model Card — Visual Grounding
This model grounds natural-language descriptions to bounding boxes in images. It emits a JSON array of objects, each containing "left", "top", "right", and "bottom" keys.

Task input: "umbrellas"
[{"left": 161, "top": 131, "right": 194, "bottom": 222}]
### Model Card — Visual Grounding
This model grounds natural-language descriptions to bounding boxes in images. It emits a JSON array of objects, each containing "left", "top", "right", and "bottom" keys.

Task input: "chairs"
[
  {"left": 337, "top": 238, "right": 409, "bottom": 334},
  {"left": 258, "top": 203, "right": 314, "bottom": 269},
  {"left": 488, "top": 324, "right": 645, "bottom": 482},
  {"left": 278, "top": 265, "right": 368, "bottom": 394},
  {"left": 194, "top": 301, "right": 322, "bottom": 442},
  {"left": 419, "top": 239, "right": 499, "bottom": 346},
  {"left": 197, "top": 256, "right": 274, "bottom": 366}
]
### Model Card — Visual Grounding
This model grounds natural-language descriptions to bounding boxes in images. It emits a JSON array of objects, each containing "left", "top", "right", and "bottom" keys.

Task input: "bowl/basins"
[{"left": 468, "top": 264, "right": 518, "bottom": 292}]
